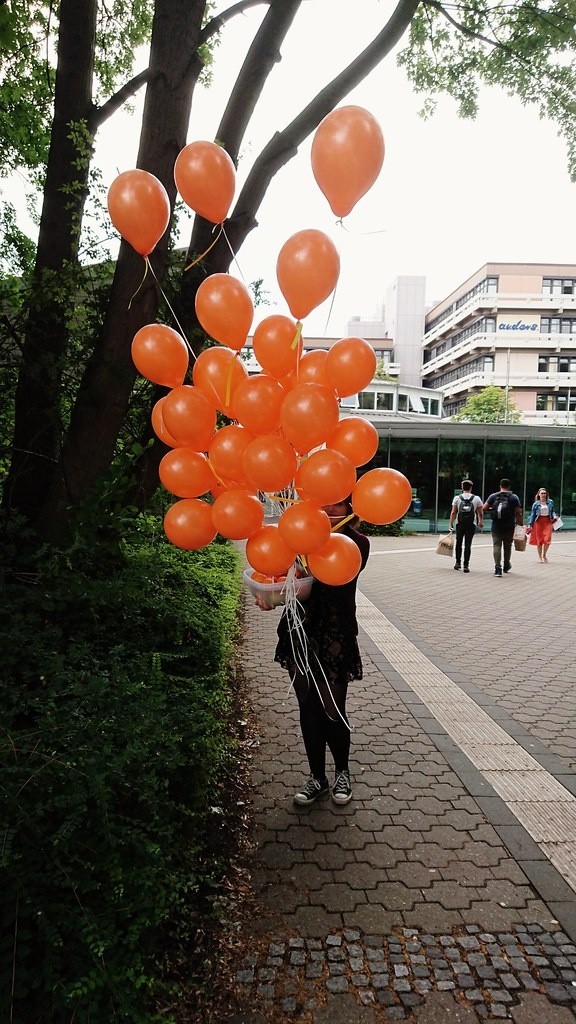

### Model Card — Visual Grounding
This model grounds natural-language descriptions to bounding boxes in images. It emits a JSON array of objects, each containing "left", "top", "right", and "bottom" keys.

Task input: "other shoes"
[
  {"left": 503, "top": 564, "right": 511, "bottom": 573},
  {"left": 454, "top": 563, "right": 461, "bottom": 569},
  {"left": 543, "top": 555, "right": 548, "bottom": 563},
  {"left": 463, "top": 567, "right": 469, "bottom": 573},
  {"left": 494, "top": 568, "right": 502, "bottom": 577},
  {"left": 537, "top": 559, "right": 543, "bottom": 563}
]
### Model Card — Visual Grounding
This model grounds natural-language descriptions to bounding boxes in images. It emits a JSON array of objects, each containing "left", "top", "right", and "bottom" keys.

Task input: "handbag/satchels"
[{"left": 435, "top": 531, "right": 453, "bottom": 557}]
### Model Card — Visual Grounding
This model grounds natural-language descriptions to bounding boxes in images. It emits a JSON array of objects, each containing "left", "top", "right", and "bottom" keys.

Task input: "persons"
[
  {"left": 449, "top": 480, "right": 484, "bottom": 573},
  {"left": 482, "top": 479, "right": 523, "bottom": 576},
  {"left": 527, "top": 489, "right": 558, "bottom": 563},
  {"left": 253, "top": 495, "right": 370, "bottom": 805}
]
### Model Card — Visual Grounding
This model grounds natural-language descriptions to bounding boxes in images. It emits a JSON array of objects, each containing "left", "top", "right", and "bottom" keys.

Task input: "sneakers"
[
  {"left": 332, "top": 770, "right": 352, "bottom": 804},
  {"left": 294, "top": 772, "right": 328, "bottom": 804}
]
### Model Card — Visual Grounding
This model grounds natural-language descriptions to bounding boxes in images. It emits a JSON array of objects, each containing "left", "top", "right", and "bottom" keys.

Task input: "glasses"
[{"left": 539, "top": 493, "right": 546, "bottom": 494}]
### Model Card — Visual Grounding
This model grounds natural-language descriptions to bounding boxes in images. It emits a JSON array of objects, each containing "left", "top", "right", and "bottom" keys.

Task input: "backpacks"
[
  {"left": 458, "top": 494, "right": 476, "bottom": 525},
  {"left": 491, "top": 492, "right": 513, "bottom": 523}
]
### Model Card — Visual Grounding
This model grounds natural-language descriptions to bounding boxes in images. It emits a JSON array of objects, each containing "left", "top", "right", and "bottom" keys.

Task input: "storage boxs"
[{"left": 244, "top": 564, "right": 315, "bottom": 606}]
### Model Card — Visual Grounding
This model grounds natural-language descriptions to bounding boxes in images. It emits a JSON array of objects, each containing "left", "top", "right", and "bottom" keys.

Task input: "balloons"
[
  {"left": 299, "top": 340, "right": 377, "bottom": 398},
  {"left": 306, "top": 533, "right": 362, "bottom": 586},
  {"left": 152, "top": 370, "right": 379, "bottom": 504},
  {"left": 244, "top": 526, "right": 295, "bottom": 575},
  {"left": 174, "top": 140, "right": 236, "bottom": 222},
  {"left": 193, "top": 348, "right": 246, "bottom": 415},
  {"left": 253, "top": 315, "right": 304, "bottom": 379},
  {"left": 352, "top": 468, "right": 412, "bottom": 524},
  {"left": 132, "top": 324, "right": 188, "bottom": 388},
  {"left": 277, "top": 229, "right": 341, "bottom": 319},
  {"left": 107, "top": 170, "right": 170, "bottom": 254},
  {"left": 195, "top": 273, "right": 254, "bottom": 351},
  {"left": 164, "top": 500, "right": 218, "bottom": 550},
  {"left": 280, "top": 503, "right": 332, "bottom": 554},
  {"left": 310, "top": 105, "right": 385, "bottom": 217},
  {"left": 210, "top": 491, "right": 264, "bottom": 540}
]
